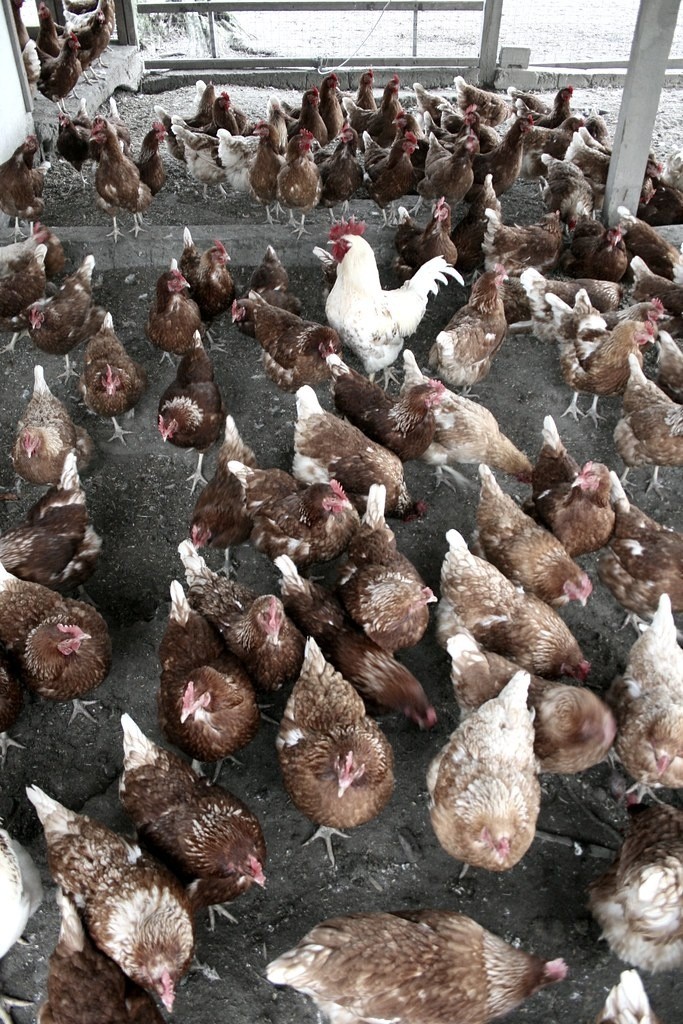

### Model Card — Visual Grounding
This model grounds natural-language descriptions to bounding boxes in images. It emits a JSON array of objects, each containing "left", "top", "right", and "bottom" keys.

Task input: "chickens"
[{"left": 1, "top": 0, "right": 683, "bottom": 1023}]
